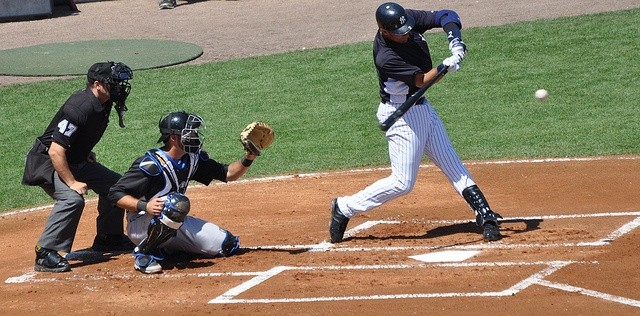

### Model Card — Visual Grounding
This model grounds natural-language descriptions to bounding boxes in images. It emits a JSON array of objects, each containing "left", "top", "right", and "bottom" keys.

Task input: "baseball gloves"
[{"left": 240, "top": 122, "right": 275, "bottom": 157}]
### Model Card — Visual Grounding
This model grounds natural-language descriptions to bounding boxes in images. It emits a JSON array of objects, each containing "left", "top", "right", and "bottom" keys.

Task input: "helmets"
[
  {"left": 87, "top": 61, "right": 137, "bottom": 127},
  {"left": 376, "top": 3, "right": 416, "bottom": 35},
  {"left": 157, "top": 112, "right": 207, "bottom": 154}
]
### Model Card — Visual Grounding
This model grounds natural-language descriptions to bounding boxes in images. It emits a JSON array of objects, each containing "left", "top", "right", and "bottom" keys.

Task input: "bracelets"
[
  {"left": 440, "top": 23, "right": 463, "bottom": 40},
  {"left": 432, "top": 61, "right": 449, "bottom": 80},
  {"left": 135, "top": 199, "right": 151, "bottom": 214},
  {"left": 239, "top": 153, "right": 255, "bottom": 169}
]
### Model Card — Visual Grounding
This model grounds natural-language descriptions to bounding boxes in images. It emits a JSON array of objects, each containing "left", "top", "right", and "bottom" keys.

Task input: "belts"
[{"left": 382, "top": 97, "right": 425, "bottom": 104}]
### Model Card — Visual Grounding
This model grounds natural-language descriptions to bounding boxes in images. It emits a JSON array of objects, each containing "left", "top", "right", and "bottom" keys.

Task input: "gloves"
[
  {"left": 443, "top": 55, "right": 461, "bottom": 72},
  {"left": 449, "top": 38, "right": 466, "bottom": 61}
]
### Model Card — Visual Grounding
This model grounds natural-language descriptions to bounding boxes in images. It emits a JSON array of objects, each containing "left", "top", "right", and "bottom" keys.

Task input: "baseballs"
[{"left": 535, "top": 89, "right": 548, "bottom": 101}]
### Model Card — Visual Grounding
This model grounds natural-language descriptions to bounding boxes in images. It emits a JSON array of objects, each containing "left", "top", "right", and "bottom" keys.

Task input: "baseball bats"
[{"left": 381, "top": 49, "right": 468, "bottom": 132}]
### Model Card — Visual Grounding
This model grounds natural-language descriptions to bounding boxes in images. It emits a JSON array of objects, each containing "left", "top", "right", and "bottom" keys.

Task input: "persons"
[
  {"left": 327, "top": 1, "right": 502, "bottom": 242},
  {"left": 19, "top": 57, "right": 133, "bottom": 273},
  {"left": 105, "top": 108, "right": 259, "bottom": 274}
]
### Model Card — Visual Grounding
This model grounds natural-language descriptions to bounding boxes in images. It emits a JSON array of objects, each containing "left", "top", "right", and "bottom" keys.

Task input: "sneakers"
[
  {"left": 34, "top": 246, "right": 71, "bottom": 272},
  {"left": 93, "top": 234, "right": 136, "bottom": 251},
  {"left": 329, "top": 198, "right": 349, "bottom": 243},
  {"left": 159, "top": 0, "right": 177, "bottom": 8},
  {"left": 483, "top": 220, "right": 499, "bottom": 242},
  {"left": 134, "top": 259, "right": 162, "bottom": 274}
]
